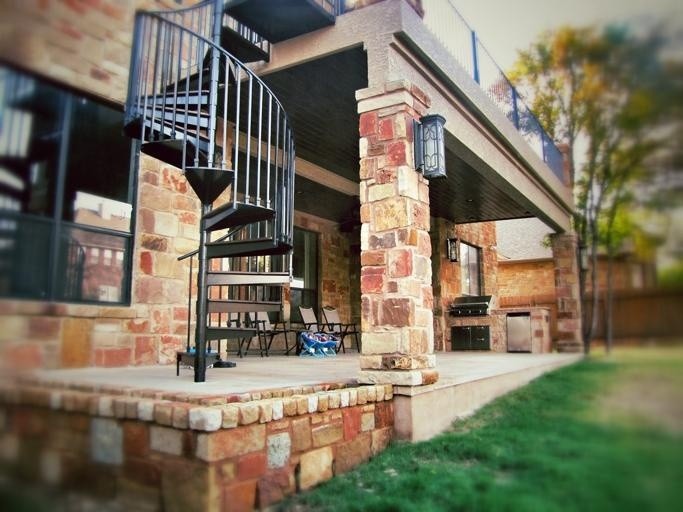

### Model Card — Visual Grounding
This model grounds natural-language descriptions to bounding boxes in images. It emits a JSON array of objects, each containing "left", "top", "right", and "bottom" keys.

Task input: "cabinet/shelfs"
[{"left": 449, "top": 295, "right": 495, "bottom": 350}]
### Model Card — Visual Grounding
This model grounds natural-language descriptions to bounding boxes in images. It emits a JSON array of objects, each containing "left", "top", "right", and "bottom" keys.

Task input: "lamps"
[
  {"left": 414, "top": 114, "right": 446, "bottom": 179},
  {"left": 447, "top": 237, "right": 459, "bottom": 262}
]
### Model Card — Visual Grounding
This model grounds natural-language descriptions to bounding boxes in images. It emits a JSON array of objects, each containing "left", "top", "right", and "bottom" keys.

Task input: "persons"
[{"left": 308, "top": 332, "right": 338, "bottom": 342}]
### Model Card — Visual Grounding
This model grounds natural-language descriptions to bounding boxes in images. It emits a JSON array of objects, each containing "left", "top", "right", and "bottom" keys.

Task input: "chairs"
[
  {"left": 320, "top": 306, "right": 359, "bottom": 353},
  {"left": 244, "top": 306, "right": 294, "bottom": 355},
  {"left": 298, "top": 305, "right": 338, "bottom": 356},
  {"left": 229, "top": 311, "right": 269, "bottom": 358}
]
